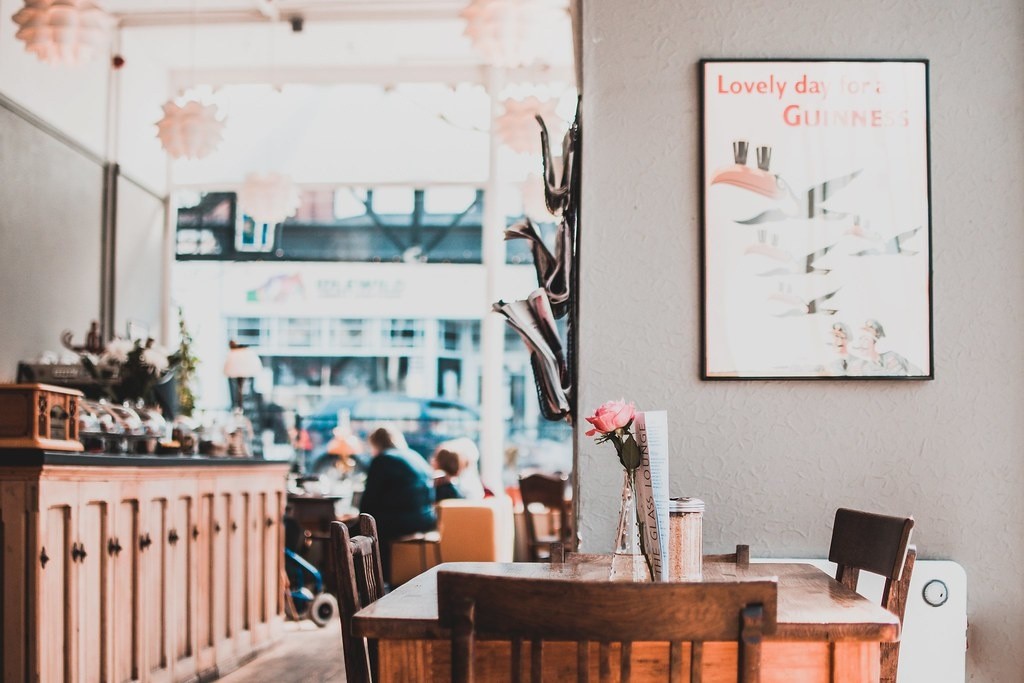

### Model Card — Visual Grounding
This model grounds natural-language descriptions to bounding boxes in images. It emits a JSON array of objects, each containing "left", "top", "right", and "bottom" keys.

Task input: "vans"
[{"left": 298, "top": 392, "right": 486, "bottom": 482}]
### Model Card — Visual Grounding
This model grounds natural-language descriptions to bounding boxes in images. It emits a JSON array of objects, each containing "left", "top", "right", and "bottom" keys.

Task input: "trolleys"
[{"left": 283, "top": 544, "right": 338, "bottom": 628}]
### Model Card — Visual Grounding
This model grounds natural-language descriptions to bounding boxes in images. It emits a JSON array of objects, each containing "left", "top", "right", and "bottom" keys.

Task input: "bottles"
[{"left": 668, "top": 497, "right": 707, "bottom": 579}]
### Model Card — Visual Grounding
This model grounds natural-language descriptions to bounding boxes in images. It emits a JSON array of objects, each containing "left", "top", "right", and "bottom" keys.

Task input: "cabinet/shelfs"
[{"left": 1, "top": 446, "right": 288, "bottom": 683}]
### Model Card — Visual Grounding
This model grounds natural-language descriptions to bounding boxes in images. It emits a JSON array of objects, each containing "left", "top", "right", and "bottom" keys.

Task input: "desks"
[
  {"left": 350, "top": 563, "right": 903, "bottom": 683},
  {"left": 288, "top": 482, "right": 368, "bottom": 595},
  {"left": 513, "top": 501, "right": 573, "bottom": 561}
]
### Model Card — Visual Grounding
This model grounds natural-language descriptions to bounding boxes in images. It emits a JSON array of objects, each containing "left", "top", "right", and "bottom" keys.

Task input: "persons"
[
  {"left": 357, "top": 425, "right": 438, "bottom": 599},
  {"left": 424, "top": 441, "right": 468, "bottom": 513}
]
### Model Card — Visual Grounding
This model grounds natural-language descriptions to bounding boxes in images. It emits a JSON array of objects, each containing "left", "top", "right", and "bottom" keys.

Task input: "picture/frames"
[{"left": 698, "top": 58, "right": 935, "bottom": 380}]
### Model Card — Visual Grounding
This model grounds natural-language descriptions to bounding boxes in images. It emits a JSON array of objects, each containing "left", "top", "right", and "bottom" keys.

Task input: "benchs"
[{"left": 390, "top": 493, "right": 516, "bottom": 587}]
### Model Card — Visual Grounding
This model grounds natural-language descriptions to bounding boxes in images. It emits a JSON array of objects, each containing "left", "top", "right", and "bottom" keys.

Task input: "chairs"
[
  {"left": 325, "top": 515, "right": 388, "bottom": 683},
  {"left": 702, "top": 545, "right": 750, "bottom": 563},
  {"left": 519, "top": 474, "right": 573, "bottom": 562},
  {"left": 828, "top": 507, "right": 916, "bottom": 683},
  {"left": 438, "top": 572, "right": 777, "bottom": 682}
]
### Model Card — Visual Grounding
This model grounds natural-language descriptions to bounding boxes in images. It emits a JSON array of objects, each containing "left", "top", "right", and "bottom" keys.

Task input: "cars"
[{"left": 502, "top": 435, "right": 577, "bottom": 496}]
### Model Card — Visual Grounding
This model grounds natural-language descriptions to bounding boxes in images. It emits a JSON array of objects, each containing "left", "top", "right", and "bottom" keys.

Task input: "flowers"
[{"left": 586, "top": 399, "right": 657, "bottom": 583}]
